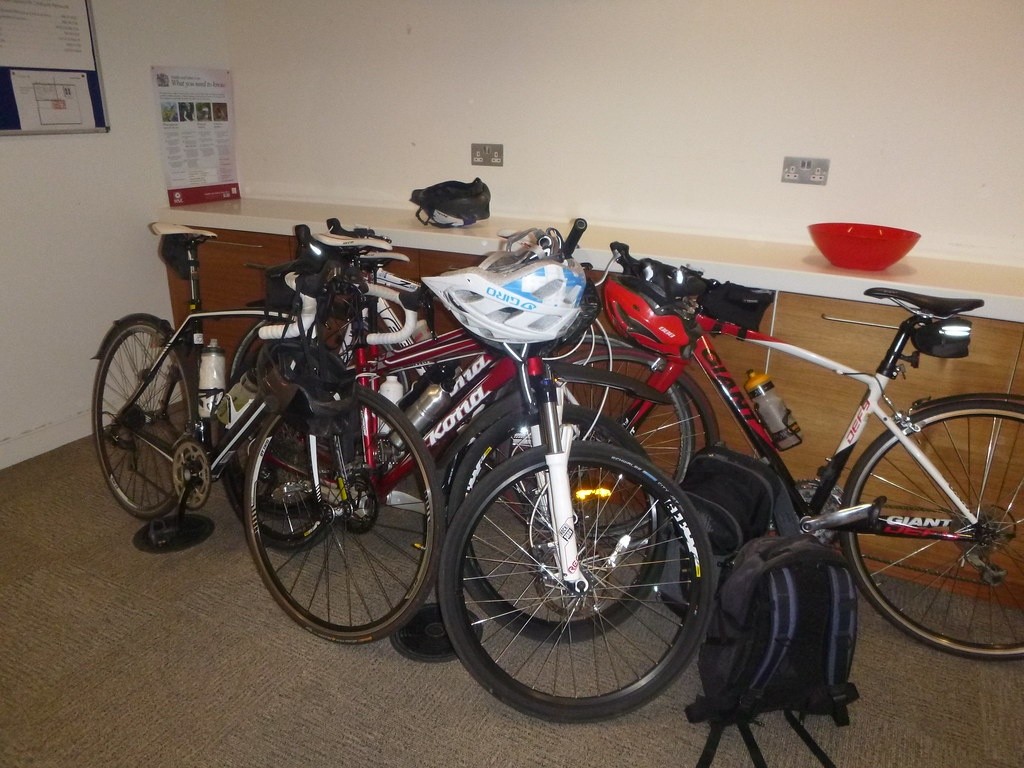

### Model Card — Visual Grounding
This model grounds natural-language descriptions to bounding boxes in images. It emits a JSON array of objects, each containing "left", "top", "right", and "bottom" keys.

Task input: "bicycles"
[
  {"left": 218, "top": 210, "right": 714, "bottom": 726},
  {"left": 85, "top": 222, "right": 451, "bottom": 646},
  {"left": 497, "top": 244, "right": 1024, "bottom": 663}
]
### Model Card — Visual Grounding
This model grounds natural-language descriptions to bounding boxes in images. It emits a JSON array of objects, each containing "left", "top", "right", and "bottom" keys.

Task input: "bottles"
[
  {"left": 388, "top": 376, "right": 455, "bottom": 449},
  {"left": 744, "top": 370, "right": 805, "bottom": 451},
  {"left": 196, "top": 332, "right": 227, "bottom": 419},
  {"left": 216, "top": 364, "right": 268, "bottom": 423},
  {"left": 378, "top": 372, "right": 403, "bottom": 437}
]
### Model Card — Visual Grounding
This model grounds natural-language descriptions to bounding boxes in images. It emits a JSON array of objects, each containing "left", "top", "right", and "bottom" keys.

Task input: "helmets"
[
  {"left": 605, "top": 272, "right": 703, "bottom": 358},
  {"left": 410, "top": 178, "right": 491, "bottom": 228},
  {"left": 255, "top": 339, "right": 359, "bottom": 417},
  {"left": 421, "top": 258, "right": 586, "bottom": 342}
]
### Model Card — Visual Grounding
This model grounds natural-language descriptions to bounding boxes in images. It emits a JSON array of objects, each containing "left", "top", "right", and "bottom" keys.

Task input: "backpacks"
[
  {"left": 657, "top": 446, "right": 802, "bottom": 621},
  {"left": 686, "top": 536, "right": 860, "bottom": 768}
]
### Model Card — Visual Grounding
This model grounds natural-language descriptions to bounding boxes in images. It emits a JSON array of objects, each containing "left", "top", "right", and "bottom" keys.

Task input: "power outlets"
[
  {"left": 471, "top": 144, "right": 503, "bottom": 166},
  {"left": 781, "top": 156, "right": 830, "bottom": 186}
]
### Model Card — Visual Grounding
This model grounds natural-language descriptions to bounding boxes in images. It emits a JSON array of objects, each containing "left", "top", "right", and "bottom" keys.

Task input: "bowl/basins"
[{"left": 808, "top": 222, "right": 923, "bottom": 272}]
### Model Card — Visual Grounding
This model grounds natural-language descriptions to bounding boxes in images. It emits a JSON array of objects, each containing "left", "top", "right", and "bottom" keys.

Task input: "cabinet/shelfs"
[{"left": 162, "top": 196, "right": 1024, "bottom": 605}]
